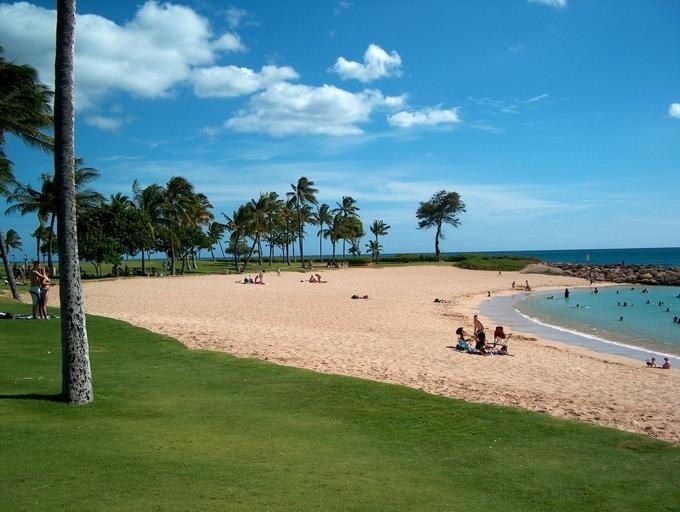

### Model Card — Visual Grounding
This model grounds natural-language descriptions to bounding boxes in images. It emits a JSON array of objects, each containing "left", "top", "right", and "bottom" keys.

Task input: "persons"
[
  {"left": 526, "top": 280, "right": 532, "bottom": 292},
  {"left": 13, "top": 262, "right": 25, "bottom": 279},
  {"left": 310, "top": 274, "right": 321, "bottom": 283},
  {"left": 112, "top": 265, "right": 161, "bottom": 276},
  {"left": 512, "top": 281, "right": 516, "bottom": 288},
  {"left": 244, "top": 270, "right": 266, "bottom": 284},
  {"left": 661, "top": 357, "right": 670, "bottom": 369},
  {"left": 472, "top": 314, "right": 487, "bottom": 355},
  {"left": 646, "top": 357, "right": 657, "bottom": 367},
  {"left": 28, "top": 260, "right": 53, "bottom": 320},
  {"left": 565, "top": 272, "right": 680, "bottom": 323}
]
